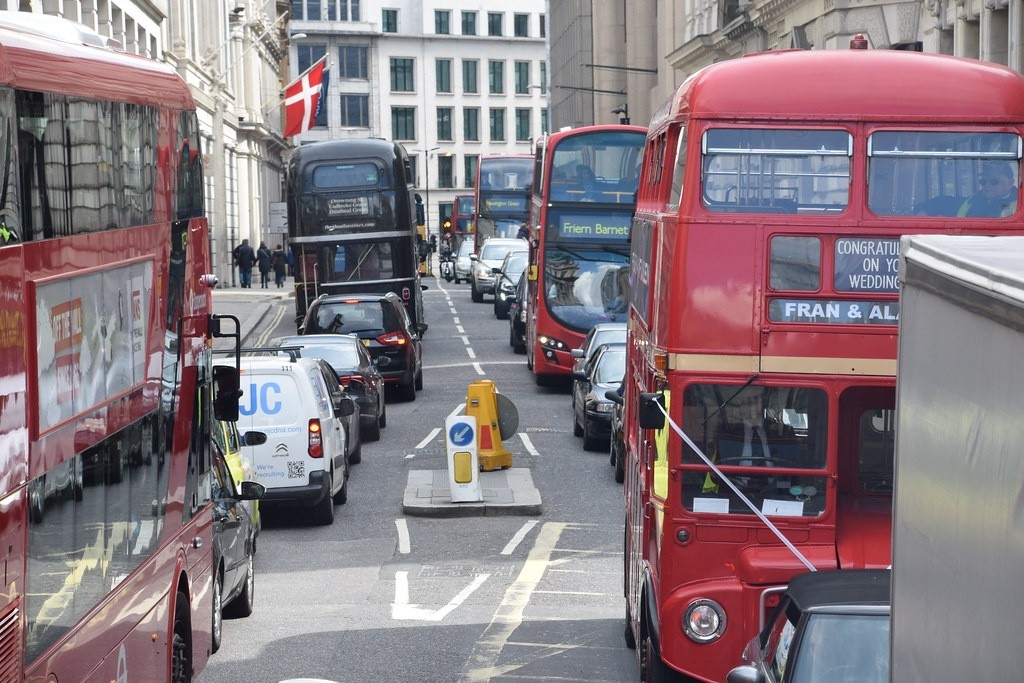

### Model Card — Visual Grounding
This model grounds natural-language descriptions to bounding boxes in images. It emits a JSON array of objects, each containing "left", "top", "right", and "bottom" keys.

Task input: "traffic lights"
[{"left": 443, "top": 217, "right": 451, "bottom": 240}]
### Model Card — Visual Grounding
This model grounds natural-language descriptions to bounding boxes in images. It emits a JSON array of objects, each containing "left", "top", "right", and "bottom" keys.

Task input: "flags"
[{"left": 283, "top": 60, "right": 328, "bottom": 140}]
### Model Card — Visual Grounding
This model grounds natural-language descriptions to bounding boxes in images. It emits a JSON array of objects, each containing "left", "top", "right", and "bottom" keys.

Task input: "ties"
[{"left": 751, "top": 425, "right": 769, "bottom": 484}]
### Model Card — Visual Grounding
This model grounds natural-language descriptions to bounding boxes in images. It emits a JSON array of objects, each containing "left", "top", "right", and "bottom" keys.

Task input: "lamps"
[
  {"left": 232, "top": 6, "right": 244, "bottom": 13},
  {"left": 276, "top": 33, "right": 307, "bottom": 43}
]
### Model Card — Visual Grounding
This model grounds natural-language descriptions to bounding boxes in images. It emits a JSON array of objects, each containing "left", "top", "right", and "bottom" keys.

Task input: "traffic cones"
[{"left": 464, "top": 381, "right": 514, "bottom": 473}]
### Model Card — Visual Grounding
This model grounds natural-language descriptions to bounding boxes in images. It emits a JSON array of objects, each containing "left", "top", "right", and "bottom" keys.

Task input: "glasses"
[{"left": 978, "top": 177, "right": 999, "bottom": 185}]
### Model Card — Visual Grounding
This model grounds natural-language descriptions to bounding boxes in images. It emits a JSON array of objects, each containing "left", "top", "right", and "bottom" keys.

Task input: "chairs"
[{"left": 363, "top": 309, "right": 379, "bottom": 319}]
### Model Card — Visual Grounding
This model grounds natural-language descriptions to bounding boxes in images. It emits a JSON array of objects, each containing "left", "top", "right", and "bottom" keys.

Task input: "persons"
[
  {"left": 518, "top": 223, "right": 530, "bottom": 239},
  {"left": 232, "top": 240, "right": 293, "bottom": 288},
  {"left": 438, "top": 246, "right": 455, "bottom": 273},
  {"left": 713, "top": 384, "right": 812, "bottom": 500},
  {"left": 481, "top": 169, "right": 499, "bottom": 189},
  {"left": 551, "top": 166, "right": 642, "bottom": 201}
]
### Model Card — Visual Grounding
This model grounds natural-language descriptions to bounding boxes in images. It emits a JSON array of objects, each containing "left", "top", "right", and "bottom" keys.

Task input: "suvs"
[
  {"left": 296, "top": 291, "right": 430, "bottom": 402},
  {"left": 211, "top": 343, "right": 356, "bottom": 525},
  {"left": 469, "top": 236, "right": 529, "bottom": 304}
]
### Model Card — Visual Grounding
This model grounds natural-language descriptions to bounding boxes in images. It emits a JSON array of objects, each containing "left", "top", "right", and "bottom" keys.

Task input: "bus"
[
  {"left": 473, "top": 151, "right": 538, "bottom": 262},
  {"left": 0, "top": 10, "right": 245, "bottom": 683},
  {"left": 622, "top": 48, "right": 1024, "bottom": 683},
  {"left": 452, "top": 194, "right": 476, "bottom": 240},
  {"left": 526, "top": 124, "right": 650, "bottom": 388},
  {"left": 278, "top": 138, "right": 433, "bottom": 342}
]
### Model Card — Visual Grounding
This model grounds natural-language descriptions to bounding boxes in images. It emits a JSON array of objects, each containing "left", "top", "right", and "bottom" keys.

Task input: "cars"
[
  {"left": 491, "top": 250, "right": 530, "bottom": 320},
  {"left": 450, "top": 241, "right": 476, "bottom": 283},
  {"left": 724, "top": 566, "right": 892, "bottom": 683},
  {"left": 570, "top": 341, "right": 628, "bottom": 452},
  {"left": 211, "top": 437, "right": 267, "bottom": 652},
  {"left": 212, "top": 420, "right": 269, "bottom": 535},
  {"left": 505, "top": 267, "right": 528, "bottom": 355},
  {"left": 569, "top": 323, "right": 628, "bottom": 410},
  {"left": 603, "top": 373, "right": 627, "bottom": 485},
  {"left": 311, "top": 358, "right": 367, "bottom": 481},
  {"left": 267, "top": 332, "right": 391, "bottom": 444}
]
